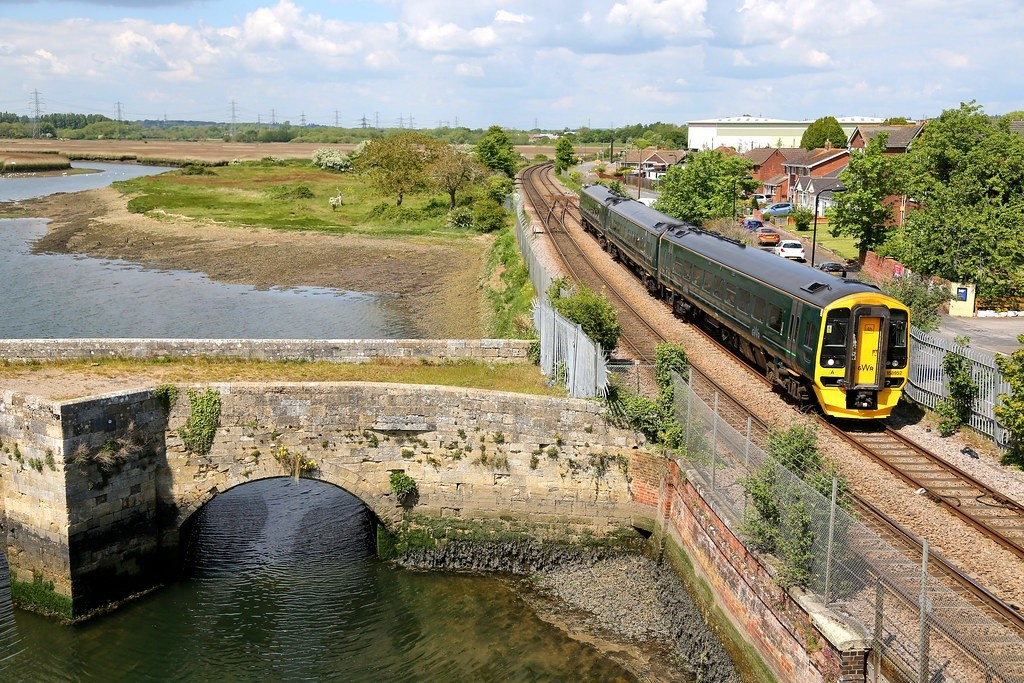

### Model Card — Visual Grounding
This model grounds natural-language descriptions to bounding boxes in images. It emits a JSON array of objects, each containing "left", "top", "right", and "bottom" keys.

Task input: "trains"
[{"left": 578, "top": 184, "right": 909, "bottom": 423}]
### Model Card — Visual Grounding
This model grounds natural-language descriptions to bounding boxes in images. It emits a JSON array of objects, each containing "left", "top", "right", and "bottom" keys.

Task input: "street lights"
[
  {"left": 811, "top": 187, "right": 848, "bottom": 267},
  {"left": 603, "top": 140, "right": 608, "bottom": 152},
  {"left": 669, "top": 154, "right": 677, "bottom": 165}
]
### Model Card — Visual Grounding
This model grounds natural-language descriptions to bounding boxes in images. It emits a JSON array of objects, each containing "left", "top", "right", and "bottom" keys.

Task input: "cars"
[
  {"left": 758, "top": 201, "right": 793, "bottom": 221},
  {"left": 815, "top": 262, "right": 847, "bottom": 277},
  {"left": 739, "top": 189, "right": 772, "bottom": 205},
  {"left": 775, "top": 239, "right": 806, "bottom": 263},
  {"left": 736, "top": 213, "right": 764, "bottom": 230},
  {"left": 751, "top": 227, "right": 781, "bottom": 246}
]
